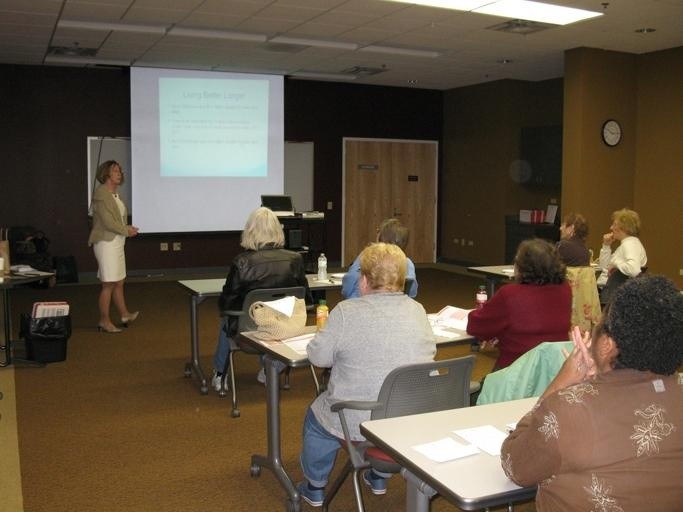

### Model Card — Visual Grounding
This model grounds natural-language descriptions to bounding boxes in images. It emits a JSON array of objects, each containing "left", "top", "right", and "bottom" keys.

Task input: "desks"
[
  {"left": 177, "top": 272, "right": 346, "bottom": 395},
  {"left": 238, "top": 310, "right": 475, "bottom": 512},
  {"left": 1, "top": 268, "right": 58, "bottom": 368},
  {"left": 467, "top": 264, "right": 516, "bottom": 302},
  {"left": 359, "top": 392, "right": 542, "bottom": 511}
]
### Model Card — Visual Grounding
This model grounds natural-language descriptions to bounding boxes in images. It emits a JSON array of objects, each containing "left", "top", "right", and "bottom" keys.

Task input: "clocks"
[{"left": 602, "top": 120, "right": 623, "bottom": 146}]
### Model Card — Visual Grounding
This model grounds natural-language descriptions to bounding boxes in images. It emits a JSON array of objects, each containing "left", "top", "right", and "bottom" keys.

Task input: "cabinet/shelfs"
[{"left": 504, "top": 215, "right": 559, "bottom": 263}]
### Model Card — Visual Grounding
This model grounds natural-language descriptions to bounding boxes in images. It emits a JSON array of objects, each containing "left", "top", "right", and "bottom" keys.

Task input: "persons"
[
  {"left": 501, "top": 280, "right": 683, "bottom": 512},
  {"left": 212, "top": 207, "right": 312, "bottom": 395},
  {"left": 340, "top": 216, "right": 418, "bottom": 301},
  {"left": 292, "top": 243, "right": 437, "bottom": 507},
  {"left": 87, "top": 160, "right": 139, "bottom": 333},
  {"left": 464, "top": 238, "right": 572, "bottom": 374},
  {"left": 552, "top": 211, "right": 591, "bottom": 267},
  {"left": 595, "top": 209, "right": 648, "bottom": 296}
]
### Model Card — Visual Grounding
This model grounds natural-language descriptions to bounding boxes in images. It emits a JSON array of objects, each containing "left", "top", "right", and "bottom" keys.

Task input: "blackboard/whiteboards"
[{"left": 88, "top": 136, "right": 315, "bottom": 218}]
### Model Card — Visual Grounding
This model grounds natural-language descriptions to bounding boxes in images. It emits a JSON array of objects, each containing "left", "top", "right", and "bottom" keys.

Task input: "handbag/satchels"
[{"left": 247, "top": 294, "right": 308, "bottom": 342}]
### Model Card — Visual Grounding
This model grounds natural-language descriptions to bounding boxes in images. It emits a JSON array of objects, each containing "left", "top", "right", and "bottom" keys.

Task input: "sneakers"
[
  {"left": 294, "top": 479, "right": 326, "bottom": 508},
  {"left": 363, "top": 469, "right": 387, "bottom": 495},
  {"left": 211, "top": 371, "right": 229, "bottom": 392},
  {"left": 257, "top": 367, "right": 268, "bottom": 387}
]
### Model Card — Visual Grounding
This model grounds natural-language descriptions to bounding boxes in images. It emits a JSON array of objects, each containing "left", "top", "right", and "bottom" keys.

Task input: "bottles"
[
  {"left": 318, "top": 252, "right": 328, "bottom": 282},
  {"left": 475, "top": 284, "right": 488, "bottom": 310},
  {"left": 316, "top": 299, "right": 329, "bottom": 337}
]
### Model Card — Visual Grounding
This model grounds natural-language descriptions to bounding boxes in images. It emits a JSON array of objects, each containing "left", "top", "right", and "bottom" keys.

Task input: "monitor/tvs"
[{"left": 261, "top": 193, "right": 294, "bottom": 215}]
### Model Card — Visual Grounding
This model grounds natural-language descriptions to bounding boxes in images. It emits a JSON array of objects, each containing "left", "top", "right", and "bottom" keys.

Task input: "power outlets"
[
  {"left": 160, "top": 243, "right": 169, "bottom": 251},
  {"left": 173, "top": 242, "right": 181, "bottom": 251}
]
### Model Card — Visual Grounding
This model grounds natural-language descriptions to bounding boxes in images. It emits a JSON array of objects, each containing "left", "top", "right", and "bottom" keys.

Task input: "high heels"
[
  {"left": 96, "top": 321, "right": 122, "bottom": 334},
  {"left": 121, "top": 311, "right": 140, "bottom": 328}
]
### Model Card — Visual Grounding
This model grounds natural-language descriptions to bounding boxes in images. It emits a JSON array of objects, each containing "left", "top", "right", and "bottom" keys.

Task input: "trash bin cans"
[{"left": 19, "top": 313, "right": 72, "bottom": 363}]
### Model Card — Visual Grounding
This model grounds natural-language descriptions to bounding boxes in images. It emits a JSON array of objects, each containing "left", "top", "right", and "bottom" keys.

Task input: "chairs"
[
  {"left": 403, "top": 278, "right": 415, "bottom": 295},
  {"left": 220, "top": 286, "right": 307, "bottom": 417},
  {"left": 601, "top": 266, "right": 649, "bottom": 307},
  {"left": 319, "top": 354, "right": 477, "bottom": 512}
]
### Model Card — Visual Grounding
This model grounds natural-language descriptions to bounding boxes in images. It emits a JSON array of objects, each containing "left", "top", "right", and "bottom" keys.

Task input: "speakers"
[{"left": 519, "top": 123, "right": 563, "bottom": 187}]
